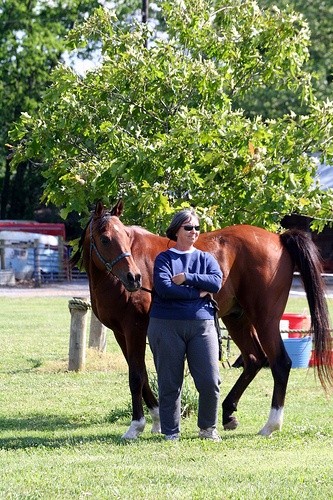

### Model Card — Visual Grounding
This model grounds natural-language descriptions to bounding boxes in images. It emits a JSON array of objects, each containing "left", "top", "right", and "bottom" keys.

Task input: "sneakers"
[
  {"left": 199, "top": 425, "right": 222, "bottom": 441},
  {"left": 165, "top": 433, "right": 179, "bottom": 441}
]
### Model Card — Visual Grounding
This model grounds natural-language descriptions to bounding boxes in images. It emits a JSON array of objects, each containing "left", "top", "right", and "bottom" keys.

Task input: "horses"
[{"left": 63, "top": 200, "right": 333, "bottom": 439}]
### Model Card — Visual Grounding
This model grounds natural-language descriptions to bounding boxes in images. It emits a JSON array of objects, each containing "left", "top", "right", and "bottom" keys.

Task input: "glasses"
[{"left": 181, "top": 225, "right": 200, "bottom": 231}]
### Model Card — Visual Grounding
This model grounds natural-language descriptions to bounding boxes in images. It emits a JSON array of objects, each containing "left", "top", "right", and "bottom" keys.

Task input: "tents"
[{"left": 0, "top": 221, "right": 66, "bottom": 281}]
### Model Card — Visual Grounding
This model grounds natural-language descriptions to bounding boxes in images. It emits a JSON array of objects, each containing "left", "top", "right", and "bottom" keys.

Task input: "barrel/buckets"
[
  {"left": 283, "top": 337, "right": 312, "bottom": 369},
  {"left": 279, "top": 314, "right": 307, "bottom": 340}
]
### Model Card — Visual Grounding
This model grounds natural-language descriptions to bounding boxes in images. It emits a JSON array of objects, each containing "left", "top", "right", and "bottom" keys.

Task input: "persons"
[{"left": 148, "top": 211, "right": 223, "bottom": 441}]
